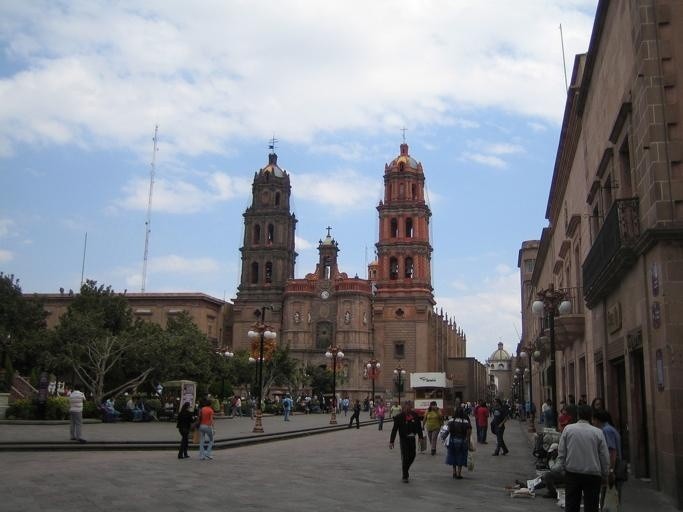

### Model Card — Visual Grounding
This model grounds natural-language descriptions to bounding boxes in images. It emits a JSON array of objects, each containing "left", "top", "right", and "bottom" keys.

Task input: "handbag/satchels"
[
  {"left": 192, "top": 432, "right": 200, "bottom": 443},
  {"left": 418, "top": 429, "right": 427, "bottom": 451},
  {"left": 602, "top": 472, "right": 619, "bottom": 512}
]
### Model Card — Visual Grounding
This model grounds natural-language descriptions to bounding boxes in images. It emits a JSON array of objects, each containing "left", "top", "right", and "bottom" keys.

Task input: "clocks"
[{"left": 321, "top": 290, "right": 329, "bottom": 300}]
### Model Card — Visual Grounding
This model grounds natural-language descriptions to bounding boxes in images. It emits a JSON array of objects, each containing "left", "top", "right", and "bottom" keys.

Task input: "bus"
[{"left": 408, "top": 371, "right": 454, "bottom": 420}]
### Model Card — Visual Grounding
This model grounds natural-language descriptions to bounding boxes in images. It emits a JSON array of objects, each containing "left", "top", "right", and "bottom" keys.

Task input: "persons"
[
  {"left": 476, "top": 399, "right": 509, "bottom": 456},
  {"left": 192, "top": 404, "right": 199, "bottom": 446},
  {"left": 198, "top": 399, "right": 215, "bottom": 462},
  {"left": 66, "top": 388, "right": 73, "bottom": 396},
  {"left": 135, "top": 393, "right": 144, "bottom": 413},
  {"left": 283, "top": 396, "right": 337, "bottom": 422},
  {"left": 343, "top": 397, "right": 402, "bottom": 431},
  {"left": 126, "top": 396, "right": 142, "bottom": 421},
  {"left": 104, "top": 395, "right": 120, "bottom": 423},
  {"left": 174, "top": 400, "right": 197, "bottom": 459},
  {"left": 423, "top": 401, "right": 472, "bottom": 479},
  {"left": 230, "top": 396, "right": 272, "bottom": 417},
  {"left": 69, "top": 383, "right": 87, "bottom": 442},
  {"left": 389, "top": 400, "right": 423, "bottom": 483},
  {"left": 460, "top": 398, "right": 536, "bottom": 419},
  {"left": 539, "top": 396, "right": 622, "bottom": 511},
  {"left": 63, "top": 385, "right": 69, "bottom": 397}
]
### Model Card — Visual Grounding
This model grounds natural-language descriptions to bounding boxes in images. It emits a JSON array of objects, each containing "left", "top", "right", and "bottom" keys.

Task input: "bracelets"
[{"left": 212, "top": 429, "right": 215, "bottom": 433}]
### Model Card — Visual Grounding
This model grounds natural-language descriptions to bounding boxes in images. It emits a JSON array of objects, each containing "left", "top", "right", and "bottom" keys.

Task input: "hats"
[{"left": 548, "top": 443, "right": 558, "bottom": 452}]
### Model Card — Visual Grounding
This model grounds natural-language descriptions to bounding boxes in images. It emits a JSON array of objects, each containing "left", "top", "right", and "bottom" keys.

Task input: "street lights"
[
  {"left": 366, "top": 351, "right": 381, "bottom": 419},
  {"left": 531, "top": 283, "right": 571, "bottom": 433},
  {"left": 392, "top": 359, "right": 405, "bottom": 405},
  {"left": 510, "top": 363, "right": 528, "bottom": 422},
  {"left": 520, "top": 341, "right": 540, "bottom": 433},
  {"left": 214, "top": 345, "right": 233, "bottom": 401},
  {"left": 247, "top": 307, "right": 276, "bottom": 432},
  {"left": 325, "top": 334, "right": 344, "bottom": 424}
]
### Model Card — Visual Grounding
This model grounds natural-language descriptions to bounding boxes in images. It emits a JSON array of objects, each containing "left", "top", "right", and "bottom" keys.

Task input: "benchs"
[{"left": 101, "top": 408, "right": 146, "bottom": 422}]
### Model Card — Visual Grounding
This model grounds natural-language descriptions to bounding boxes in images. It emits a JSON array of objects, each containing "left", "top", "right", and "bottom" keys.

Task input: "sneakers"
[{"left": 402, "top": 475, "right": 408, "bottom": 483}]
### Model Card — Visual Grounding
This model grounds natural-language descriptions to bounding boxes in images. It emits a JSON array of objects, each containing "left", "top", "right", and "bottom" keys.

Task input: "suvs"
[{"left": 362, "top": 394, "right": 384, "bottom": 410}]
